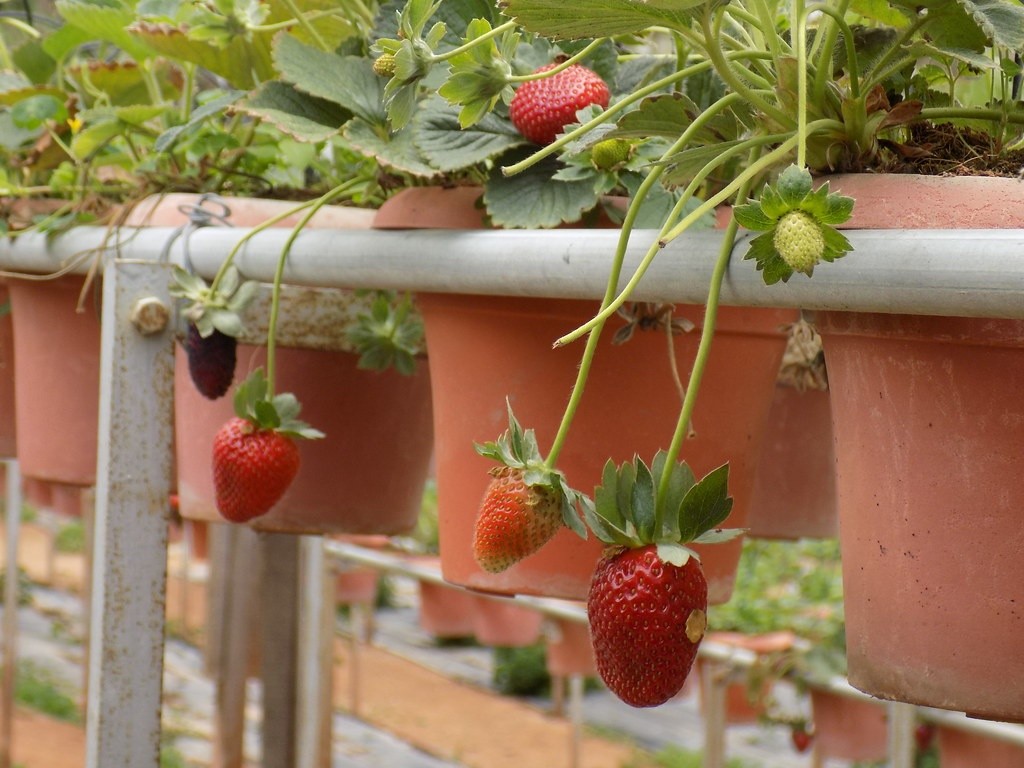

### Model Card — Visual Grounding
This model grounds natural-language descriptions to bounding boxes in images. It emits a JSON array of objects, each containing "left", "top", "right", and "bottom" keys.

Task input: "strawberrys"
[
  {"left": 474, "top": 468, "right": 564, "bottom": 574},
  {"left": 511, "top": 62, "right": 611, "bottom": 143},
  {"left": 211, "top": 417, "right": 301, "bottom": 523},
  {"left": 587, "top": 543, "right": 709, "bottom": 709}
]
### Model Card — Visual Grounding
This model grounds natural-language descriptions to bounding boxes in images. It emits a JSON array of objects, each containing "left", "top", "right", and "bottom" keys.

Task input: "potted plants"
[{"left": 0, "top": 2, "right": 1024, "bottom": 767}]
